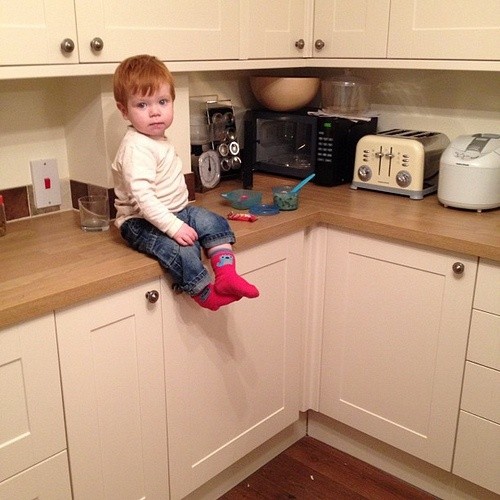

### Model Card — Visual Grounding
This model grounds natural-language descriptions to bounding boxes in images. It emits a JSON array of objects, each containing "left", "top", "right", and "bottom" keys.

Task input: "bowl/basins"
[
  {"left": 321, "top": 80, "right": 373, "bottom": 115},
  {"left": 271, "top": 185, "right": 299, "bottom": 210},
  {"left": 248, "top": 75, "right": 321, "bottom": 112},
  {"left": 225, "top": 188, "right": 262, "bottom": 211}
]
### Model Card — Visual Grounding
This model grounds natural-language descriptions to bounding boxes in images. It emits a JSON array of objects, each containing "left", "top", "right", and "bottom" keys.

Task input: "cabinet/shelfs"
[
  {"left": 0, "top": 0, "right": 315, "bottom": 81},
  {"left": 314, "top": 0, "right": 500, "bottom": 73},
  {"left": 55, "top": 228, "right": 305, "bottom": 500},
  {"left": 0, "top": 310, "right": 72, "bottom": 500},
  {"left": 311, "top": 219, "right": 500, "bottom": 500}
]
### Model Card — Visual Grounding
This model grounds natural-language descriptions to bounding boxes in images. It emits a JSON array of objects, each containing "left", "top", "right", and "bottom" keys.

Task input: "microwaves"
[{"left": 244, "top": 108, "right": 379, "bottom": 187}]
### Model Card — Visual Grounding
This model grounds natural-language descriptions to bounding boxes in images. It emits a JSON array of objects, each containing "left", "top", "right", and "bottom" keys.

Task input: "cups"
[{"left": 77, "top": 195, "right": 110, "bottom": 232}]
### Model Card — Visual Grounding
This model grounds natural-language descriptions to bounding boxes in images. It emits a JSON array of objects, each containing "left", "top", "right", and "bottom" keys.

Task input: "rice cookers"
[{"left": 437, "top": 133, "right": 500, "bottom": 212}]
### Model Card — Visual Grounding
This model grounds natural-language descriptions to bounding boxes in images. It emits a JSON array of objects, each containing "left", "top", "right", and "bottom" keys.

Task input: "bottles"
[{"left": 0, "top": 195, "right": 7, "bottom": 237}]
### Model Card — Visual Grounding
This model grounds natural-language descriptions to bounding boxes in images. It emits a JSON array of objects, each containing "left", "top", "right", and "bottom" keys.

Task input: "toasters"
[{"left": 350, "top": 128, "right": 450, "bottom": 201}]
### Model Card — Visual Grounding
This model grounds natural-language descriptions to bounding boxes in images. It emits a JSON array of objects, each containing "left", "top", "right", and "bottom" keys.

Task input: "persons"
[{"left": 112, "top": 53, "right": 258, "bottom": 312}]
